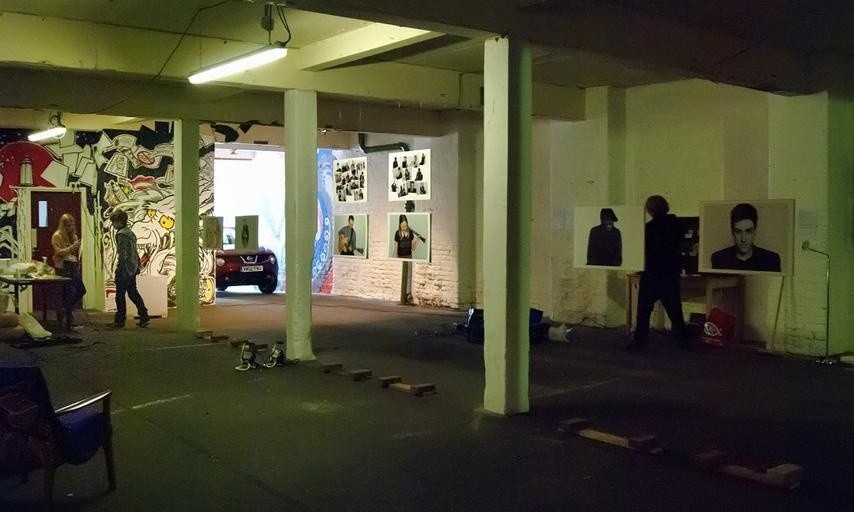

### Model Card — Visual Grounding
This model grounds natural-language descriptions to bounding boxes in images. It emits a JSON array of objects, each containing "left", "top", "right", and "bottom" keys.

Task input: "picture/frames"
[
  {"left": 330, "top": 148, "right": 432, "bottom": 263},
  {"left": 698, "top": 196, "right": 797, "bottom": 278},
  {"left": 570, "top": 201, "right": 647, "bottom": 273}
]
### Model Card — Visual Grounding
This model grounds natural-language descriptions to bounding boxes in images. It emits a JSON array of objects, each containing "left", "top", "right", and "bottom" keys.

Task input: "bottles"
[
  {"left": 17, "top": 156, "right": 33, "bottom": 187},
  {"left": 815, "top": 358, "right": 831, "bottom": 364}
]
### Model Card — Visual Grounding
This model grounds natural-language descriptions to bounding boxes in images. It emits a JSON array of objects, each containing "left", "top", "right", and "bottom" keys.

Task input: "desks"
[{"left": 0, "top": 273, "right": 75, "bottom": 333}]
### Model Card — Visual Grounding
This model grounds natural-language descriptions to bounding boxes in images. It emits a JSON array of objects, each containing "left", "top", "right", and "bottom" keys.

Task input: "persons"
[
  {"left": 710, "top": 203, "right": 781, "bottom": 271},
  {"left": 106, "top": 210, "right": 150, "bottom": 329},
  {"left": 625, "top": 195, "right": 690, "bottom": 350},
  {"left": 390, "top": 154, "right": 426, "bottom": 196},
  {"left": 52, "top": 213, "right": 86, "bottom": 329},
  {"left": 241, "top": 219, "right": 249, "bottom": 245},
  {"left": 335, "top": 160, "right": 365, "bottom": 200},
  {"left": 587, "top": 208, "right": 622, "bottom": 266},
  {"left": 338, "top": 215, "right": 356, "bottom": 256},
  {"left": 395, "top": 215, "right": 422, "bottom": 258}
]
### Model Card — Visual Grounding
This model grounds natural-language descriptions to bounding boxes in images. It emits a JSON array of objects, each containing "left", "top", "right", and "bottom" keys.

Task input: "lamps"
[
  {"left": 25, "top": 113, "right": 73, "bottom": 147},
  {"left": 182, "top": 1, "right": 296, "bottom": 89}
]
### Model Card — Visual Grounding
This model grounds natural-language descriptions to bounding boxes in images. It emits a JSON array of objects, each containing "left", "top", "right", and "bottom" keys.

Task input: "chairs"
[{"left": 0, "top": 362, "right": 119, "bottom": 506}]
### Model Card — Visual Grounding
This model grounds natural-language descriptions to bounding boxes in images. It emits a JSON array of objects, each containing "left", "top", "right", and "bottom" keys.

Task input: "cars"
[{"left": 216, "top": 225, "right": 277, "bottom": 293}]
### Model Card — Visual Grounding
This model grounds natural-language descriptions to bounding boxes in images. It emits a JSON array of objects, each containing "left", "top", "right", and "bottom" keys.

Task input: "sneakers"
[
  {"left": 56, "top": 307, "right": 65, "bottom": 324},
  {"left": 136, "top": 319, "right": 152, "bottom": 329},
  {"left": 66, "top": 315, "right": 79, "bottom": 327},
  {"left": 104, "top": 321, "right": 126, "bottom": 330}
]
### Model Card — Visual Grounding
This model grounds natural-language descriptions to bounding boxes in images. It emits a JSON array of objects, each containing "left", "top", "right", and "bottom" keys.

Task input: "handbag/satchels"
[{"left": 697, "top": 308, "right": 735, "bottom": 349}]
